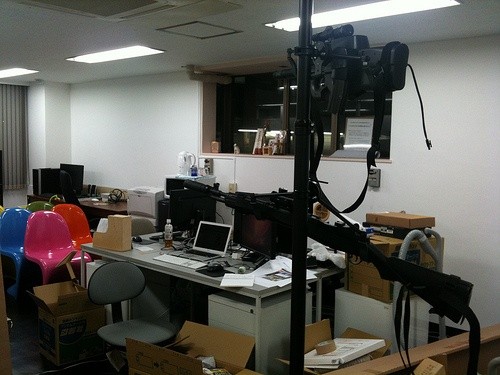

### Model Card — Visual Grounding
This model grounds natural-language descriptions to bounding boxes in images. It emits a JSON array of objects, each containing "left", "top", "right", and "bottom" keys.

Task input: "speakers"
[{"left": 87, "top": 184, "right": 96, "bottom": 196}]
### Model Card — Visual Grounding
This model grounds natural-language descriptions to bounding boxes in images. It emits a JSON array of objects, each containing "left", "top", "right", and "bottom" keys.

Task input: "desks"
[
  {"left": 80, "top": 232, "right": 338, "bottom": 375},
  {"left": 78, "top": 197, "right": 127, "bottom": 212}
]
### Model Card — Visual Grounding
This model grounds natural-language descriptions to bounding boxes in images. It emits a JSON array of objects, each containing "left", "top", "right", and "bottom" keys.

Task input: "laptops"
[{"left": 170, "top": 220, "right": 232, "bottom": 262}]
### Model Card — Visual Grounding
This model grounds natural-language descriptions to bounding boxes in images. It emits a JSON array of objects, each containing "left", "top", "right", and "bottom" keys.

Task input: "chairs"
[
  {"left": 0, "top": 201, "right": 102, "bottom": 289},
  {"left": 88, "top": 261, "right": 179, "bottom": 375}
]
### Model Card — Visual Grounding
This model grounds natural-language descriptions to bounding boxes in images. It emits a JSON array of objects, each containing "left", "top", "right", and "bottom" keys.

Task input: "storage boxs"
[
  {"left": 125, "top": 320, "right": 264, "bottom": 375},
  {"left": 348, "top": 212, "right": 444, "bottom": 304},
  {"left": 273, "top": 318, "right": 447, "bottom": 375},
  {"left": 27, "top": 281, "right": 105, "bottom": 369}
]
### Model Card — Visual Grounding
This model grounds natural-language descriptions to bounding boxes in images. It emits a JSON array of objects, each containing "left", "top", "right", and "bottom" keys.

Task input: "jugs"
[{"left": 176, "top": 151, "right": 196, "bottom": 176}]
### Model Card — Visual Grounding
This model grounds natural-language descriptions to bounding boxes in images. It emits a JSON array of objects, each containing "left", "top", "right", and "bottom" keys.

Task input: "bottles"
[{"left": 164, "top": 219, "right": 173, "bottom": 248}]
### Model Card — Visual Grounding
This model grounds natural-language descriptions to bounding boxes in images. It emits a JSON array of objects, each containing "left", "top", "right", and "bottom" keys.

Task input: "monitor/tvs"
[
  {"left": 231, "top": 191, "right": 276, "bottom": 260},
  {"left": 167, "top": 189, "right": 216, "bottom": 234}
]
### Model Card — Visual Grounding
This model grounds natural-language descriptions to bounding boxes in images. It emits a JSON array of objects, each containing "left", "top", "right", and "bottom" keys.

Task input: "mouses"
[
  {"left": 208, "top": 264, "right": 223, "bottom": 273},
  {"left": 132, "top": 236, "right": 142, "bottom": 242}
]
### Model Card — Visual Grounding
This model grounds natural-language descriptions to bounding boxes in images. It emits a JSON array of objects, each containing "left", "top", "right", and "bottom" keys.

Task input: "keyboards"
[{"left": 149, "top": 231, "right": 182, "bottom": 240}]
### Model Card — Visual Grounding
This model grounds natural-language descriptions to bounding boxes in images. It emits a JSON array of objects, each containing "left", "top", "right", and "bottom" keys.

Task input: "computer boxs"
[
  {"left": 32, "top": 169, "right": 60, "bottom": 196},
  {"left": 128, "top": 187, "right": 164, "bottom": 237}
]
